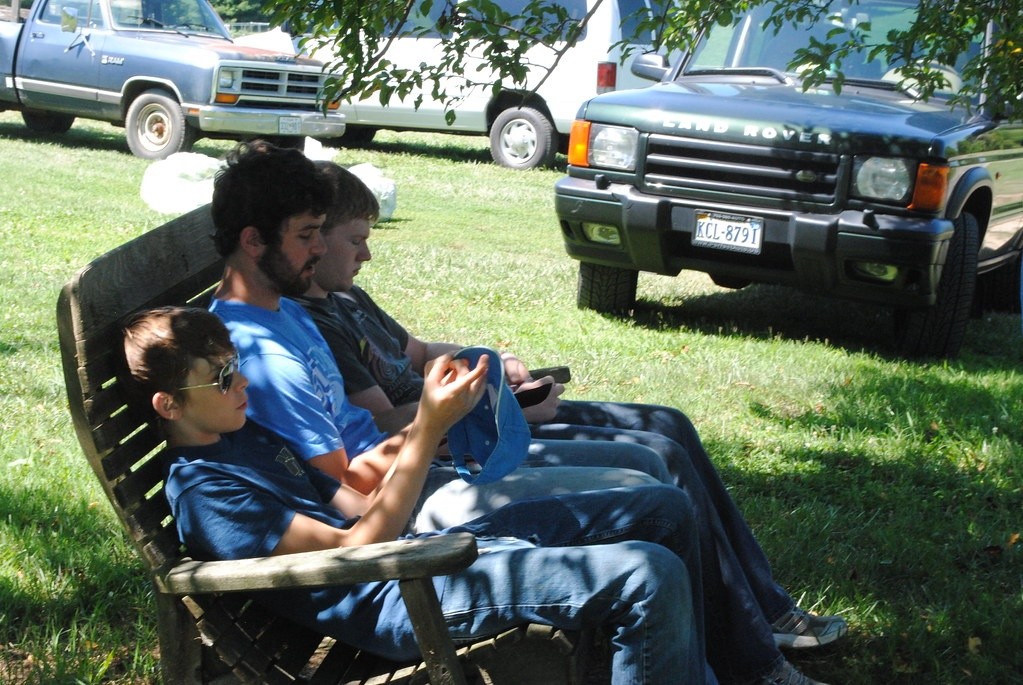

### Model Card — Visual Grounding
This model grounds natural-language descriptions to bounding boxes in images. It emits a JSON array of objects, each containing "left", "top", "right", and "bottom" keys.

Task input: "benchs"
[{"left": 56, "top": 201, "right": 610, "bottom": 683}]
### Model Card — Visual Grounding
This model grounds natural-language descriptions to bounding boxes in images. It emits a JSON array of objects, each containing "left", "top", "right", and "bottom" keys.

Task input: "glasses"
[{"left": 177, "top": 352, "right": 240, "bottom": 396}]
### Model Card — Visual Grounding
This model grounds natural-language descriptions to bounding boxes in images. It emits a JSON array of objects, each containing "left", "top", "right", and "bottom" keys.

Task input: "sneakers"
[
  {"left": 757, "top": 653, "right": 829, "bottom": 685},
  {"left": 772, "top": 603, "right": 848, "bottom": 648}
]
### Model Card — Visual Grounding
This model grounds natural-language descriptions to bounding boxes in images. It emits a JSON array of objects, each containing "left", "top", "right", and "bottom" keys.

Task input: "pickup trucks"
[{"left": 0, "top": 1, "right": 346, "bottom": 161}]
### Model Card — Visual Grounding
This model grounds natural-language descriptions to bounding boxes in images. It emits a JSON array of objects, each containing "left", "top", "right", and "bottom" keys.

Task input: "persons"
[
  {"left": 113, "top": 306, "right": 709, "bottom": 685},
  {"left": 207, "top": 139, "right": 719, "bottom": 685},
  {"left": 284, "top": 159, "right": 849, "bottom": 685}
]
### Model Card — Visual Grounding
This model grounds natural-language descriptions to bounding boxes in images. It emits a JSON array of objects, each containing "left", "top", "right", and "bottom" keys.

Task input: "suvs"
[{"left": 556, "top": 0, "right": 1023, "bottom": 364}]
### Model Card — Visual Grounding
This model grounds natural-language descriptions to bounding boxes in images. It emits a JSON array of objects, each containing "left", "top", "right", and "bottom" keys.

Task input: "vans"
[{"left": 231, "top": 0, "right": 688, "bottom": 169}]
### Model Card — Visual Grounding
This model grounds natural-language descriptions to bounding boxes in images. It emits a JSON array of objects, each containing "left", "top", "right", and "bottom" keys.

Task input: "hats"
[{"left": 446, "top": 346, "right": 530, "bottom": 485}]
